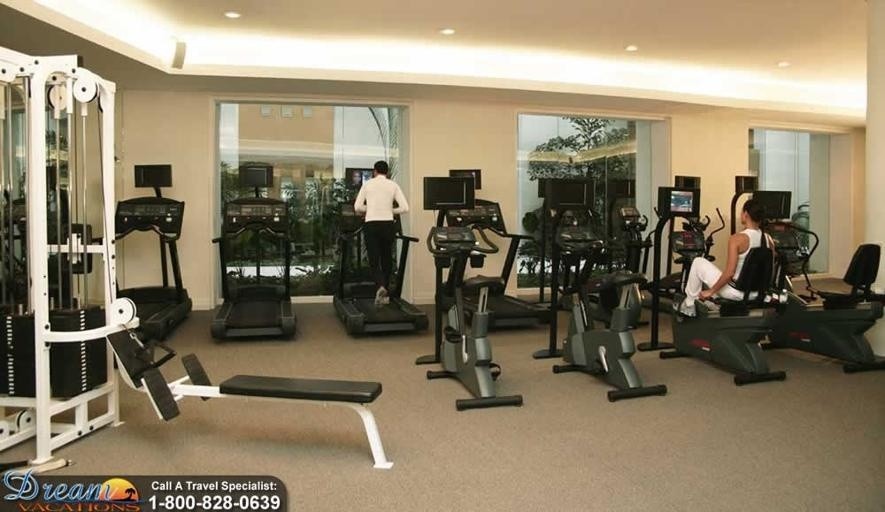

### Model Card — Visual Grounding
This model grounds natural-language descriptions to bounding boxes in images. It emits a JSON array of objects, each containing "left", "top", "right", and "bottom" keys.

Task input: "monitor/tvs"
[
  {"left": 735, "top": 176, "right": 758, "bottom": 192},
  {"left": 754, "top": 191, "right": 791, "bottom": 218},
  {"left": 450, "top": 170, "right": 481, "bottom": 189},
  {"left": 135, "top": 164, "right": 172, "bottom": 187},
  {"left": 239, "top": 166, "right": 273, "bottom": 187},
  {"left": 552, "top": 178, "right": 594, "bottom": 209},
  {"left": 675, "top": 176, "right": 700, "bottom": 188},
  {"left": 346, "top": 168, "right": 376, "bottom": 189},
  {"left": 658, "top": 186, "right": 700, "bottom": 217},
  {"left": 424, "top": 177, "right": 474, "bottom": 209}
]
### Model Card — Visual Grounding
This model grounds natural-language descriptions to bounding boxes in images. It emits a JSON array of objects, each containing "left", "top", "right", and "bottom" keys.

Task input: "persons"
[
  {"left": 673, "top": 199, "right": 777, "bottom": 318},
  {"left": 353, "top": 160, "right": 410, "bottom": 305},
  {"left": 350, "top": 170, "right": 362, "bottom": 186}
]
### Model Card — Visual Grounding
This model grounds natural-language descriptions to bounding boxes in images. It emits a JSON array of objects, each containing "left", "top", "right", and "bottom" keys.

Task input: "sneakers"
[
  {"left": 372, "top": 286, "right": 391, "bottom": 306},
  {"left": 672, "top": 301, "right": 696, "bottom": 317}
]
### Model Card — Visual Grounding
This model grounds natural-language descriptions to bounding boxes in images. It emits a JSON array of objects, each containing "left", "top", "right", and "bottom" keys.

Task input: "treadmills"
[
  {"left": 435, "top": 170, "right": 552, "bottom": 331},
  {"left": 211, "top": 162, "right": 297, "bottom": 341},
  {"left": 116, "top": 165, "right": 192, "bottom": 338},
  {"left": 323, "top": 168, "right": 429, "bottom": 337}
]
